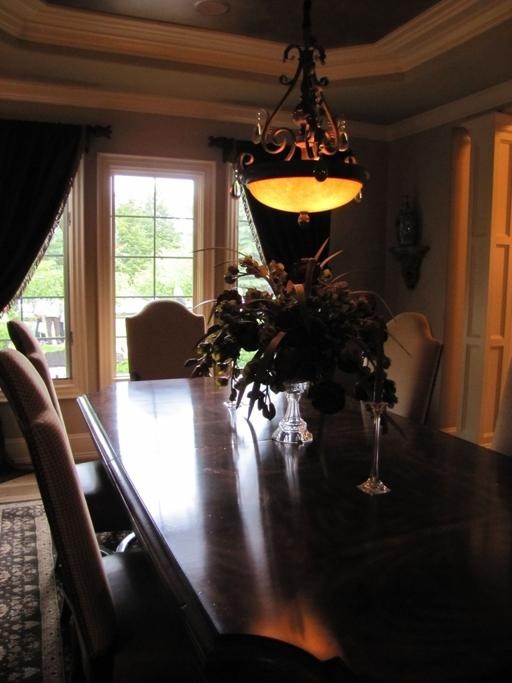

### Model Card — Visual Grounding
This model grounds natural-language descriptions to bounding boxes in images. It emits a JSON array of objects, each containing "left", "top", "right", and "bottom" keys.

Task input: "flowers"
[{"left": 182, "top": 240, "right": 394, "bottom": 427}]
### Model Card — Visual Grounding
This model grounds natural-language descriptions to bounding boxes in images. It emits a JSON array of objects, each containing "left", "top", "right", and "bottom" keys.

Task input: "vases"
[{"left": 264, "top": 371, "right": 319, "bottom": 447}]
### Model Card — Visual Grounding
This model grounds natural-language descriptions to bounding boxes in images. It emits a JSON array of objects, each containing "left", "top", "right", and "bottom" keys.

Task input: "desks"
[{"left": 79, "top": 366, "right": 512, "bottom": 683}]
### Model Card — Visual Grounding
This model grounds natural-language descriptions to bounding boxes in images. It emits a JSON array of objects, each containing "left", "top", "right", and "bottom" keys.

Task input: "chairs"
[{"left": 362, "top": 313, "right": 442, "bottom": 423}]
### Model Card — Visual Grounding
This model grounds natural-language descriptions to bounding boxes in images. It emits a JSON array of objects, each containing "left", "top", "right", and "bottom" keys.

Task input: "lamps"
[{"left": 227, "top": 3, "right": 372, "bottom": 231}]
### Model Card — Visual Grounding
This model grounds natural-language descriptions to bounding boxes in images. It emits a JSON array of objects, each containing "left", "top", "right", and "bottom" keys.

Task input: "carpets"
[{"left": 2, "top": 495, "right": 70, "bottom": 682}]
